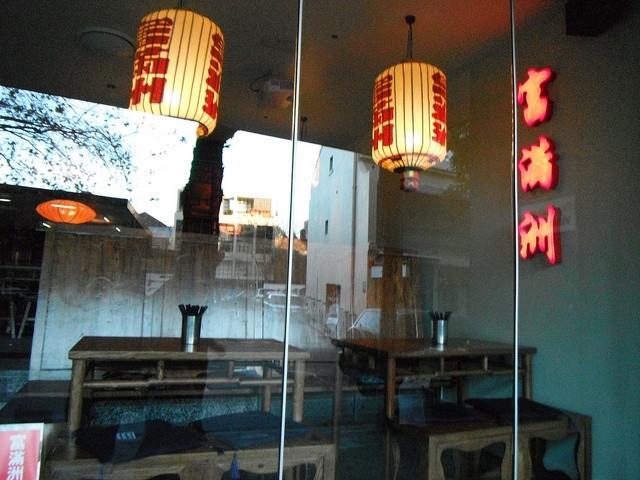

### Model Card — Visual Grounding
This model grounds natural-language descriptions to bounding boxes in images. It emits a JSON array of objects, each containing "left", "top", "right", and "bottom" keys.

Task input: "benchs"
[
  {"left": 395, "top": 396, "right": 592, "bottom": 480},
  {"left": 10, "top": 374, "right": 459, "bottom": 414},
  {"left": 45, "top": 428, "right": 336, "bottom": 480}
]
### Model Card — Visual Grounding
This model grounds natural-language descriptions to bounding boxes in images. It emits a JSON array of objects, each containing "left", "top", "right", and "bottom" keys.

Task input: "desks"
[
  {"left": 69, "top": 337, "right": 310, "bottom": 480},
  {"left": 331, "top": 337, "right": 537, "bottom": 479}
]
